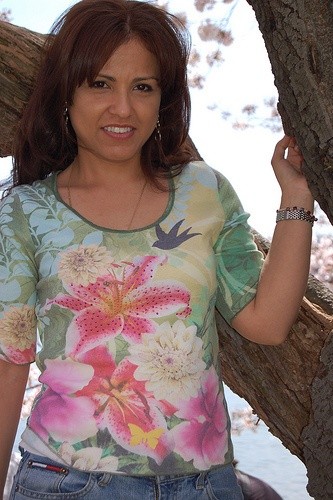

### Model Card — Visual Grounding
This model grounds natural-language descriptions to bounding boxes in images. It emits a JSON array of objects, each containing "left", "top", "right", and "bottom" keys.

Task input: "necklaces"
[{"left": 66, "top": 164, "right": 147, "bottom": 232}]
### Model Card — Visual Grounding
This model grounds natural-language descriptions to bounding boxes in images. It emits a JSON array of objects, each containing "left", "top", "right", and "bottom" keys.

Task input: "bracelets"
[{"left": 274, "top": 206, "right": 318, "bottom": 226}]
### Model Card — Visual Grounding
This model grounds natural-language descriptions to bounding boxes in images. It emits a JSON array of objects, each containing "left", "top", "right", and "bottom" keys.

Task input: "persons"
[{"left": 0, "top": 0, "right": 316, "bottom": 500}]
